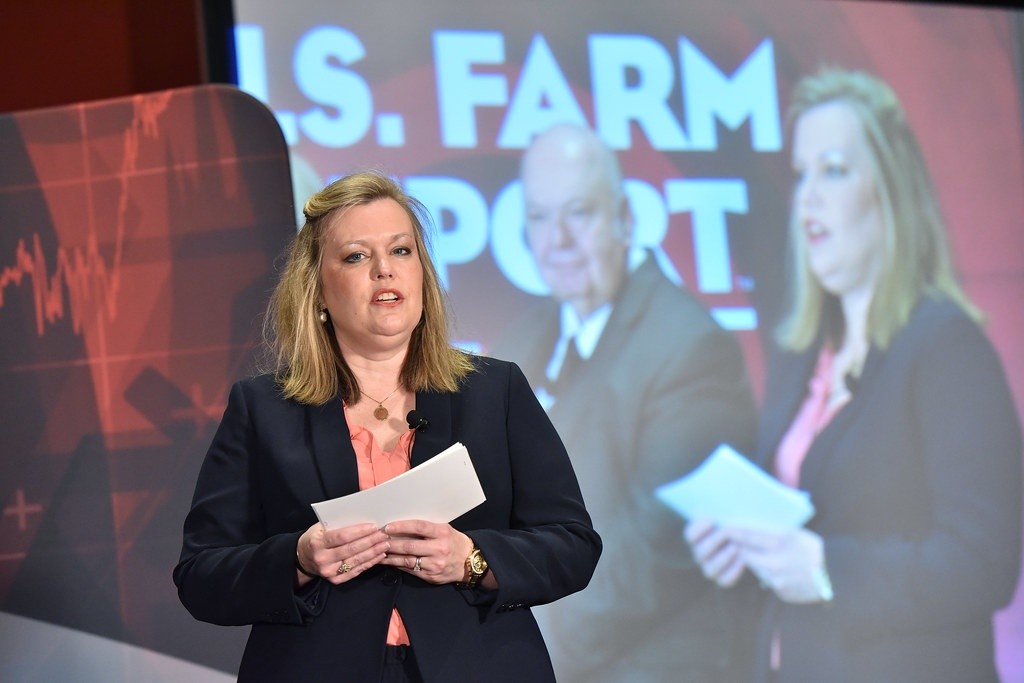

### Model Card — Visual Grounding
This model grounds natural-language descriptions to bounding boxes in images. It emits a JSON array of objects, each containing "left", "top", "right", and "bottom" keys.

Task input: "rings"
[
  {"left": 414, "top": 557, "right": 421, "bottom": 571},
  {"left": 338, "top": 560, "right": 351, "bottom": 573}
]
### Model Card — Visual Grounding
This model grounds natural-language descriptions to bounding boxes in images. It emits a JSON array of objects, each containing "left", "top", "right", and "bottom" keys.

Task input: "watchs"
[
  {"left": 817, "top": 567, "right": 833, "bottom": 602},
  {"left": 466, "top": 546, "right": 489, "bottom": 586}
]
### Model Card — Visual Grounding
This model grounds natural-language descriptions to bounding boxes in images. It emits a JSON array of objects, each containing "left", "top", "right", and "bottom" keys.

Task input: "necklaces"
[{"left": 360, "top": 383, "right": 404, "bottom": 420}]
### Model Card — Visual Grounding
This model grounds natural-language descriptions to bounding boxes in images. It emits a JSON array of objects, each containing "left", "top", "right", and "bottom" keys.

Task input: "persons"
[
  {"left": 680, "top": 69, "right": 1024, "bottom": 683},
  {"left": 170, "top": 172, "right": 604, "bottom": 683},
  {"left": 495, "top": 120, "right": 758, "bottom": 683}
]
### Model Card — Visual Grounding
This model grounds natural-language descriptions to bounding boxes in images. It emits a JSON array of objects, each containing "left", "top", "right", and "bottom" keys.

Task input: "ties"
[{"left": 553, "top": 335, "right": 588, "bottom": 402}]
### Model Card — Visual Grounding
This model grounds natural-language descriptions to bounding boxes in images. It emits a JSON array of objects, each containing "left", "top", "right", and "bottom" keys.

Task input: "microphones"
[
  {"left": 844, "top": 373, "right": 862, "bottom": 394},
  {"left": 405, "top": 410, "right": 429, "bottom": 431}
]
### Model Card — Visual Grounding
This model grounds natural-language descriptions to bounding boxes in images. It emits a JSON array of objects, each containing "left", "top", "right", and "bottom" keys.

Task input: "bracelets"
[{"left": 296, "top": 552, "right": 310, "bottom": 575}]
